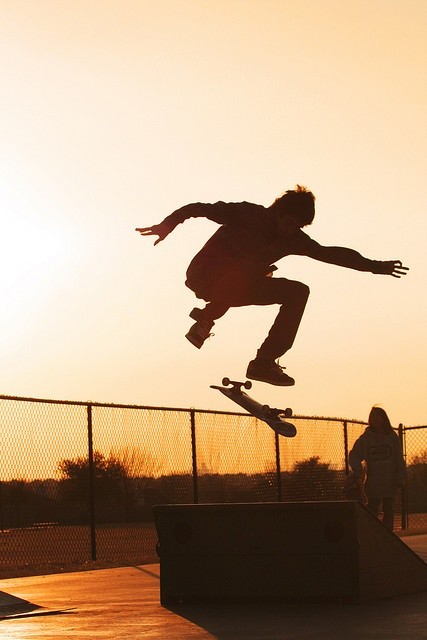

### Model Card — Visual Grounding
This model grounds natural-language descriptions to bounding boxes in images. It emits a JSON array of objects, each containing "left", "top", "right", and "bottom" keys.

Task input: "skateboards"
[{"left": 210, "top": 377, "right": 297, "bottom": 438}]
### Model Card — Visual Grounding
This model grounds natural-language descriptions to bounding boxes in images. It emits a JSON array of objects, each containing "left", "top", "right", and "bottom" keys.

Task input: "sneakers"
[
  {"left": 185, "top": 320, "right": 216, "bottom": 350},
  {"left": 245, "top": 359, "right": 295, "bottom": 386}
]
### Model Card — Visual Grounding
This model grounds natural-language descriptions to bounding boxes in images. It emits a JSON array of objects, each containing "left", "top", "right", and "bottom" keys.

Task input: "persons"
[
  {"left": 135, "top": 185, "right": 409, "bottom": 387},
  {"left": 348, "top": 408, "right": 407, "bottom": 531}
]
